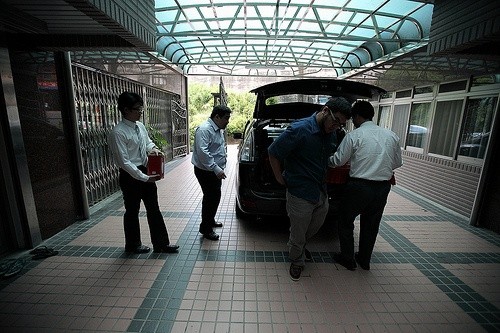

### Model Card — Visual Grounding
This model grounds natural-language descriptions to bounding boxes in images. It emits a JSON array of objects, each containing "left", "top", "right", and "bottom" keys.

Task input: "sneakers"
[
  {"left": 303, "top": 247, "right": 311, "bottom": 260},
  {"left": 289, "top": 263, "right": 303, "bottom": 281}
]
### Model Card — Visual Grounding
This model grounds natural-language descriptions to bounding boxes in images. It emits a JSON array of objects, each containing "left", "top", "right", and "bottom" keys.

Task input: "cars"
[{"left": 234, "top": 77, "right": 387, "bottom": 218}]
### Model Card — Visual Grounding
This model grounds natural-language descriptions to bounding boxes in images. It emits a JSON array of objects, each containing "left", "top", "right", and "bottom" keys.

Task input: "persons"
[
  {"left": 327, "top": 100, "right": 403, "bottom": 270},
  {"left": 267, "top": 97, "right": 352, "bottom": 280},
  {"left": 110, "top": 92, "right": 179, "bottom": 253},
  {"left": 191, "top": 105, "right": 232, "bottom": 241}
]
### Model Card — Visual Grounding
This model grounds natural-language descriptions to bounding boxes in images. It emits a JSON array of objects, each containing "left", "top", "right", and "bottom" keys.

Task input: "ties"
[{"left": 135, "top": 125, "right": 147, "bottom": 167}]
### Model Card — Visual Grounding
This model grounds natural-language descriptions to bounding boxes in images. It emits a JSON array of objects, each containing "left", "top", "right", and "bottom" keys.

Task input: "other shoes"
[
  {"left": 203, "top": 229, "right": 220, "bottom": 240},
  {"left": 334, "top": 252, "right": 357, "bottom": 270},
  {"left": 355, "top": 252, "right": 370, "bottom": 270},
  {"left": 134, "top": 245, "right": 150, "bottom": 253},
  {"left": 153, "top": 243, "right": 179, "bottom": 252},
  {"left": 213, "top": 221, "right": 222, "bottom": 228}
]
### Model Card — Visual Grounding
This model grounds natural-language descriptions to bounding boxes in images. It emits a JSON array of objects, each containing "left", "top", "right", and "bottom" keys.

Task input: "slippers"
[
  {"left": 32, "top": 250, "right": 58, "bottom": 260},
  {"left": 29, "top": 246, "right": 52, "bottom": 254}
]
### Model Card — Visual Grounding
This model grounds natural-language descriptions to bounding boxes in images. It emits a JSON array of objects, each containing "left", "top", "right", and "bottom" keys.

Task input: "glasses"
[{"left": 330, "top": 109, "right": 346, "bottom": 128}]
